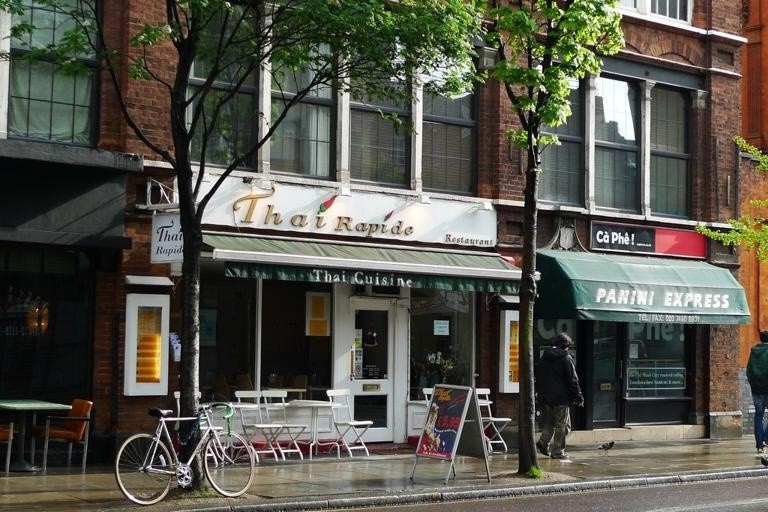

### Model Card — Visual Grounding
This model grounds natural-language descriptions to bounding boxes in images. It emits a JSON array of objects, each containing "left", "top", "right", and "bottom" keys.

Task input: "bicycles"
[{"left": 114, "top": 402, "right": 254, "bottom": 507}]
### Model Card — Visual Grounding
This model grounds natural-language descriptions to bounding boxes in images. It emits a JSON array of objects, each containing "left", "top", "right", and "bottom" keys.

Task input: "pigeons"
[{"left": 598, "top": 441, "right": 615, "bottom": 453}]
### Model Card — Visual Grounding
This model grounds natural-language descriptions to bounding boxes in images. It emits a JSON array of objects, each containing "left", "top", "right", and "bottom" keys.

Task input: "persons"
[
  {"left": 746, "top": 330, "right": 768, "bottom": 453},
  {"left": 536, "top": 333, "right": 584, "bottom": 460}
]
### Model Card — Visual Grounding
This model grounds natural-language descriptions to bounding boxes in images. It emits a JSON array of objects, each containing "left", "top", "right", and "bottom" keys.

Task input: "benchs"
[{"left": 423, "top": 386, "right": 513, "bottom": 453}]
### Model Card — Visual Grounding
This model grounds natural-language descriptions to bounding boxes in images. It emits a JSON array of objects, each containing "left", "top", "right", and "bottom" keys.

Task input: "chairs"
[
  {"left": 0, "top": 397, "right": 94, "bottom": 474},
  {"left": 208, "top": 370, "right": 375, "bottom": 460}
]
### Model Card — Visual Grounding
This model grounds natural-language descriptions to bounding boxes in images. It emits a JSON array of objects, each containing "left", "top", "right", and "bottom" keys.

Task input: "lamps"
[
  {"left": 472, "top": 199, "right": 493, "bottom": 213},
  {"left": 253, "top": 178, "right": 274, "bottom": 191},
  {"left": 332, "top": 186, "right": 350, "bottom": 197},
  {"left": 407, "top": 193, "right": 431, "bottom": 205}
]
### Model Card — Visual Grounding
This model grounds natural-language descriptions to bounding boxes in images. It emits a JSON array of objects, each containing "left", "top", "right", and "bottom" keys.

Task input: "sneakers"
[
  {"left": 756, "top": 441, "right": 768, "bottom": 454},
  {"left": 536, "top": 441, "right": 572, "bottom": 459}
]
